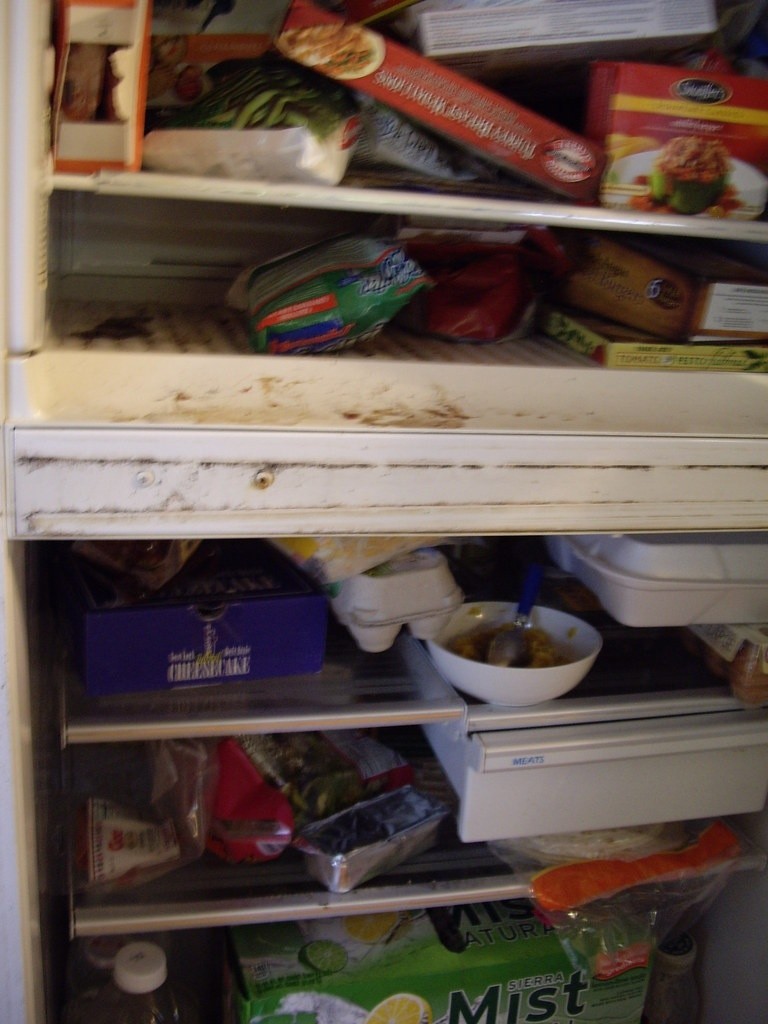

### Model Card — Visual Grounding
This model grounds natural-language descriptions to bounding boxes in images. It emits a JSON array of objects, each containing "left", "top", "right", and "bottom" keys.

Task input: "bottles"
[{"left": 69, "top": 941, "right": 210, "bottom": 1024}]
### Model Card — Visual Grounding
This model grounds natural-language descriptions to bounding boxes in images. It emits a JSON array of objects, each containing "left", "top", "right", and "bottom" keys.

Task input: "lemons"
[
  {"left": 344, "top": 912, "right": 399, "bottom": 944},
  {"left": 299, "top": 940, "right": 349, "bottom": 974}
]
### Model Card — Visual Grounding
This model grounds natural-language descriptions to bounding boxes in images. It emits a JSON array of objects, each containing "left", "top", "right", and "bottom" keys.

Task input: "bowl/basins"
[{"left": 424, "top": 600, "right": 603, "bottom": 705}]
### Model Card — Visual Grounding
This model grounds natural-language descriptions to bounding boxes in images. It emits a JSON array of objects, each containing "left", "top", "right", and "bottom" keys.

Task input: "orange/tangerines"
[{"left": 364, "top": 993, "right": 432, "bottom": 1024}]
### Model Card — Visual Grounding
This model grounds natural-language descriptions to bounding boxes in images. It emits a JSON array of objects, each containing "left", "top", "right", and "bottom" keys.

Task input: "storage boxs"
[
  {"left": 413, "top": 2, "right": 721, "bottom": 88},
  {"left": 213, "top": 892, "right": 657, "bottom": 1024},
  {"left": 53, "top": 537, "right": 332, "bottom": 700},
  {"left": 274, "top": 1, "right": 607, "bottom": 205},
  {"left": 584, "top": 58, "right": 768, "bottom": 222},
  {"left": 536, "top": 225, "right": 767, "bottom": 378},
  {"left": 46, "top": 1, "right": 154, "bottom": 177}
]
